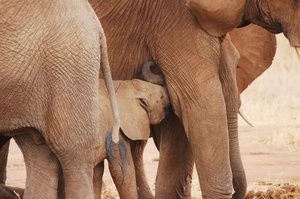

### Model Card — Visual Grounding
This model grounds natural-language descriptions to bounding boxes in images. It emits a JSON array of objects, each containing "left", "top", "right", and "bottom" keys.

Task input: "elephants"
[
  {"left": 0, "top": 0, "right": 120, "bottom": 199},
  {"left": 142, "top": 23, "right": 277, "bottom": 199},
  {"left": 93, "top": 60, "right": 173, "bottom": 199},
  {"left": 90, "top": 0, "right": 300, "bottom": 199}
]
